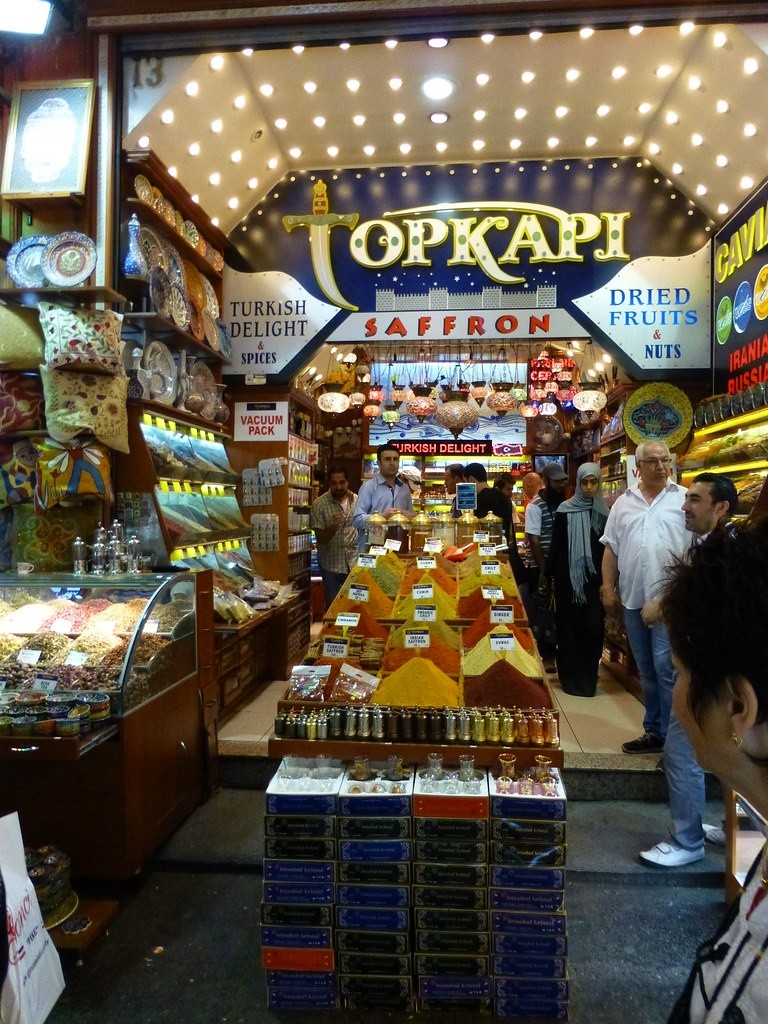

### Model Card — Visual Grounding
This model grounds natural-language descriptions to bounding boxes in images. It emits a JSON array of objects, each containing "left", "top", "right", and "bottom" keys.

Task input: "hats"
[
  {"left": 543, "top": 464, "right": 569, "bottom": 481},
  {"left": 398, "top": 465, "right": 422, "bottom": 490}
]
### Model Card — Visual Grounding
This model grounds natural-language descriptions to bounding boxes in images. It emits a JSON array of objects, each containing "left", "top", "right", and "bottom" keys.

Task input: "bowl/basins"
[{"left": 0, "top": 691, "right": 112, "bottom": 738}]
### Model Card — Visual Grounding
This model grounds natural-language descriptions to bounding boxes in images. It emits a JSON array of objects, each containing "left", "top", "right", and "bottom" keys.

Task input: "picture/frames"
[{"left": 1, "top": 78, "right": 96, "bottom": 197}]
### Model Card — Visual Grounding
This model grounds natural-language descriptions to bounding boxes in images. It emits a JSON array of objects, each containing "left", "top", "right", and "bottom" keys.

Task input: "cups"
[
  {"left": 137, "top": 555, "right": 151, "bottom": 569},
  {"left": 17, "top": 562, "right": 35, "bottom": 575}
]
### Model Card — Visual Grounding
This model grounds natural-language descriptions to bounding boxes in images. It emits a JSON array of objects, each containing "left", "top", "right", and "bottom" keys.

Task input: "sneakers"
[
  {"left": 621, "top": 732, "right": 666, "bottom": 754},
  {"left": 655, "top": 758, "right": 665, "bottom": 774}
]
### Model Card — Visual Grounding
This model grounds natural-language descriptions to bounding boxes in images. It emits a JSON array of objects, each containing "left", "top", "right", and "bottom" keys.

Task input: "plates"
[
  {"left": 143, "top": 341, "right": 178, "bottom": 404},
  {"left": 39, "top": 230, "right": 97, "bottom": 288},
  {"left": 189, "top": 361, "right": 219, "bottom": 420},
  {"left": 134, "top": 175, "right": 232, "bottom": 357},
  {"left": 5, "top": 235, "right": 53, "bottom": 288},
  {"left": 42, "top": 890, "right": 79, "bottom": 932}
]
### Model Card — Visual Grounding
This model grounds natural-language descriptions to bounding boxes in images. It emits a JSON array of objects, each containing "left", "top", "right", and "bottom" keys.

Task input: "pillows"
[{"left": 0, "top": 302, "right": 132, "bottom": 571}]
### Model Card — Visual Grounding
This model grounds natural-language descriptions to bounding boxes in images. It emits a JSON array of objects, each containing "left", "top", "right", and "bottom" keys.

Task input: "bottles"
[
  {"left": 131, "top": 348, "right": 230, "bottom": 425},
  {"left": 290, "top": 407, "right": 312, "bottom": 439},
  {"left": 488, "top": 461, "right": 532, "bottom": 473},
  {"left": 364, "top": 509, "right": 503, "bottom": 553},
  {"left": 280, "top": 751, "right": 557, "bottom": 795},
  {"left": 274, "top": 701, "right": 560, "bottom": 748},
  {"left": 119, "top": 212, "right": 147, "bottom": 278},
  {"left": 74, "top": 518, "right": 142, "bottom": 577}
]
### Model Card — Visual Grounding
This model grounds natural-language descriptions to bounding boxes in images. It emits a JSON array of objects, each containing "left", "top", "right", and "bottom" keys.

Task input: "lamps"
[{"left": 294, "top": 341, "right": 619, "bottom": 443}]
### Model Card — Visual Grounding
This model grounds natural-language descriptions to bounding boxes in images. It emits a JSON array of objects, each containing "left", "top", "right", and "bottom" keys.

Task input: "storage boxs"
[{"left": 259, "top": 755, "right": 572, "bottom": 1024}]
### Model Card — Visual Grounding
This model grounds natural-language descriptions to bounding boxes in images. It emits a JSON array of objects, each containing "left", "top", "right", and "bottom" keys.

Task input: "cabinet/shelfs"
[
  {"left": 360, "top": 453, "right": 536, "bottom": 559},
  {"left": 220, "top": 382, "right": 313, "bottom": 682},
  {"left": 570, "top": 383, "right": 768, "bottom": 747},
  {"left": 105, "top": 147, "right": 291, "bottom": 733}
]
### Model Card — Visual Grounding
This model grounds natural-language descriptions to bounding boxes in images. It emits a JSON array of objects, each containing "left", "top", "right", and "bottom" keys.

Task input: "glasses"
[
  {"left": 638, "top": 457, "right": 672, "bottom": 466},
  {"left": 409, "top": 479, "right": 423, "bottom": 487},
  {"left": 519, "top": 483, "right": 529, "bottom": 491}
]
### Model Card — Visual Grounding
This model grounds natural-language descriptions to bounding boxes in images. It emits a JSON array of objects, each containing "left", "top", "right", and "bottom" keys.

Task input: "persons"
[
  {"left": 641, "top": 473, "right": 739, "bottom": 867},
  {"left": 443, "top": 463, "right": 543, "bottom": 589},
  {"left": 525, "top": 461, "right": 571, "bottom": 672},
  {"left": 598, "top": 441, "right": 710, "bottom": 754},
  {"left": 545, "top": 463, "right": 611, "bottom": 698},
  {"left": 662, "top": 510, "right": 768, "bottom": 1024},
  {"left": 310, "top": 467, "right": 358, "bottom": 613},
  {"left": 353, "top": 444, "right": 422, "bottom": 560}
]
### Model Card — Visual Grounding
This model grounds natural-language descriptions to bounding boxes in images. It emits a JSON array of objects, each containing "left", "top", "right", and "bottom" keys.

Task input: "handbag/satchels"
[
  {"left": 529, "top": 584, "right": 549, "bottom": 628},
  {"left": 535, "top": 592, "right": 558, "bottom": 657},
  {"left": 0, "top": 812, "right": 65, "bottom": 1024}
]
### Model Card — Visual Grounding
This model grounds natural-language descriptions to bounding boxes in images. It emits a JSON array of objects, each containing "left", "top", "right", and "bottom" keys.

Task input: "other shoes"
[
  {"left": 639, "top": 840, "right": 706, "bottom": 868},
  {"left": 542, "top": 658, "right": 556, "bottom": 673},
  {"left": 702, "top": 824, "right": 728, "bottom": 847}
]
